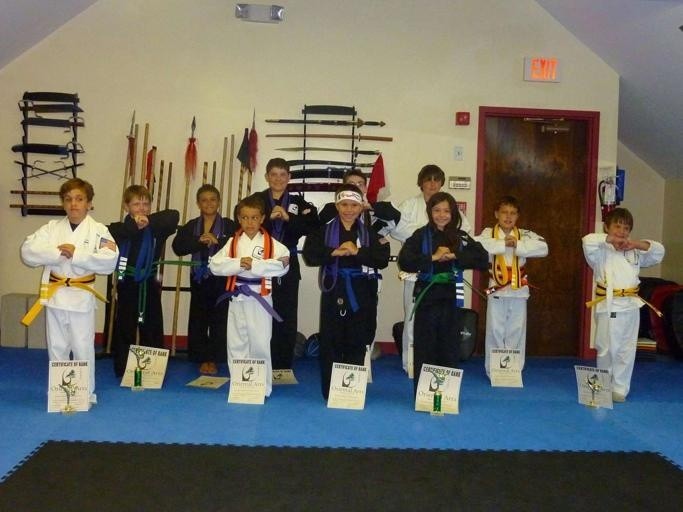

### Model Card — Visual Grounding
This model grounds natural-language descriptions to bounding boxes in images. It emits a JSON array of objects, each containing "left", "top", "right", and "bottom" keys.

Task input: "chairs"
[{"left": 636, "top": 276, "right": 683, "bottom": 362}]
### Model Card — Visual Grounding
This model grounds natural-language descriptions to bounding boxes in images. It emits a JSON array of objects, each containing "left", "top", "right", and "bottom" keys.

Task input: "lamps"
[{"left": 235, "top": 4, "right": 284, "bottom": 24}]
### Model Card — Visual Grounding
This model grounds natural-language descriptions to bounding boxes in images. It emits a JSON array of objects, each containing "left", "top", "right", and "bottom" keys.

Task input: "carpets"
[{"left": 0, "top": 439, "right": 683, "bottom": 512}]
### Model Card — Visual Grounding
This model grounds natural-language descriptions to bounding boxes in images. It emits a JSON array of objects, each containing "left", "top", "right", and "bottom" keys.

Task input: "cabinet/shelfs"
[
  {"left": 297, "top": 104, "right": 358, "bottom": 200},
  {"left": 21, "top": 90, "right": 77, "bottom": 217}
]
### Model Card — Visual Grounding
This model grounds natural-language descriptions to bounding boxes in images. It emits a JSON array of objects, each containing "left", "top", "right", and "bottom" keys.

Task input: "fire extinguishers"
[{"left": 598, "top": 176, "right": 620, "bottom": 221}]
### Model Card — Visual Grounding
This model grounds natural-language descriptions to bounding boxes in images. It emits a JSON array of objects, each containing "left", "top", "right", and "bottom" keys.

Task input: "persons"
[
  {"left": 302, "top": 183, "right": 390, "bottom": 401},
  {"left": 210, "top": 195, "right": 290, "bottom": 397},
  {"left": 398, "top": 191, "right": 489, "bottom": 399},
  {"left": 232, "top": 157, "right": 318, "bottom": 369},
  {"left": 20, "top": 177, "right": 120, "bottom": 405},
  {"left": 170, "top": 183, "right": 236, "bottom": 376},
  {"left": 317, "top": 167, "right": 401, "bottom": 382},
  {"left": 389, "top": 163, "right": 472, "bottom": 373},
  {"left": 105, "top": 184, "right": 180, "bottom": 377},
  {"left": 464, "top": 194, "right": 548, "bottom": 380},
  {"left": 581, "top": 206, "right": 666, "bottom": 403}
]
